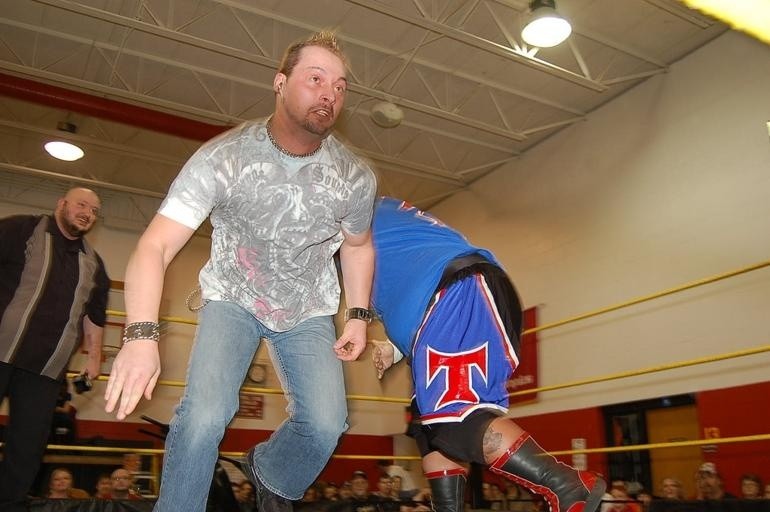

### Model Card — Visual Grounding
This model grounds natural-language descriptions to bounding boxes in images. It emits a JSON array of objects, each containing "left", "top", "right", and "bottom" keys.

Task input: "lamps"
[{"left": 520, "top": 1, "right": 573, "bottom": 49}]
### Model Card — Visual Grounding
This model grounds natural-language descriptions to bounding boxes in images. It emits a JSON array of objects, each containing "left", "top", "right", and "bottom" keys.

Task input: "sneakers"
[{"left": 240, "top": 448, "right": 293, "bottom": 512}]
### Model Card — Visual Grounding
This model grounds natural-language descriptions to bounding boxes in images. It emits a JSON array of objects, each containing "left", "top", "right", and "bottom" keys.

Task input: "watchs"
[{"left": 122, "top": 321, "right": 161, "bottom": 343}]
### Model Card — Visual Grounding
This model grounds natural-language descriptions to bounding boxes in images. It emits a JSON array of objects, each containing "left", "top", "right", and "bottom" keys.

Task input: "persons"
[
  {"left": 660, "top": 477, "right": 687, "bottom": 500},
  {"left": 335, "top": 195, "right": 607, "bottom": 510},
  {"left": 232, "top": 480, "right": 256, "bottom": 512},
  {"left": 692, "top": 461, "right": 738, "bottom": 500},
  {"left": 300, "top": 470, "right": 433, "bottom": 511},
  {"left": 103, "top": 30, "right": 378, "bottom": 510},
  {"left": 0, "top": 186, "right": 113, "bottom": 511},
  {"left": 43, "top": 467, "right": 153, "bottom": 500},
  {"left": 598, "top": 480, "right": 652, "bottom": 510},
  {"left": 740, "top": 472, "right": 770, "bottom": 500},
  {"left": 376, "top": 460, "right": 415, "bottom": 500},
  {"left": 463, "top": 481, "right": 549, "bottom": 511}
]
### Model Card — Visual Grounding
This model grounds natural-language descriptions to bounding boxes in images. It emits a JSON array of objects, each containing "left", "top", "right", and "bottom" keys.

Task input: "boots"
[
  {"left": 425, "top": 469, "right": 468, "bottom": 512},
  {"left": 488, "top": 431, "right": 607, "bottom": 511}
]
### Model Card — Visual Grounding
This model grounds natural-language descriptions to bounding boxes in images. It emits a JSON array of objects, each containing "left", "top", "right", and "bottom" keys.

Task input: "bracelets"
[{"left": 343, "top": 307, "right": 373, "bottom": 323}]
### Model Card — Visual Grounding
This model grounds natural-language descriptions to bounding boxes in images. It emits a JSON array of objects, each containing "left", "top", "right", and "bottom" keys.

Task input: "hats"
[{"left": 696, "top": 462, "right": 718, "bottom": 475}]
